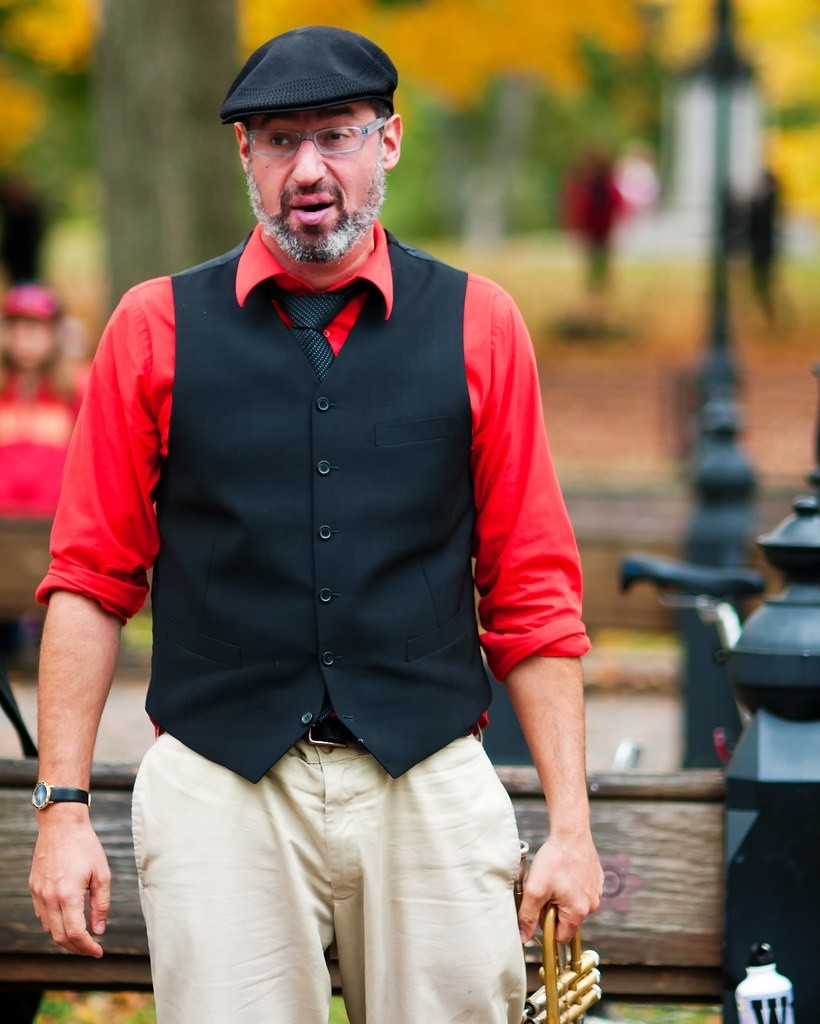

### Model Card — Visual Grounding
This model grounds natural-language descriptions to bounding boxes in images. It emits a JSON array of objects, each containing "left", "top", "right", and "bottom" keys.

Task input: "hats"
[
  {"left": 219, "top": 26, "right": 398, "bottom": 125},
  {"left": 0, "top": 284, "right": 65, "bottom": 320}
]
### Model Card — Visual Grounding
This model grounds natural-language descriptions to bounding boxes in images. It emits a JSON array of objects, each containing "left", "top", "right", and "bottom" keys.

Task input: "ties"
[{"left": 265, "top": 277, "right": 375, "bottom": 384}]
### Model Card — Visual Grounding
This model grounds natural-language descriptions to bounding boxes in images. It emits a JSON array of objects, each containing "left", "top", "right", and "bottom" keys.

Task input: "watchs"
[{"left": 30, "top": 780, "right": 92, "bottom": 811}]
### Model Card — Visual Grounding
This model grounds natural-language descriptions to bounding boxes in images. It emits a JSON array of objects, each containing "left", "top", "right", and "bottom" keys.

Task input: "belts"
[{"left": 303, "top": 707, "right": 471, "bottom": 749}]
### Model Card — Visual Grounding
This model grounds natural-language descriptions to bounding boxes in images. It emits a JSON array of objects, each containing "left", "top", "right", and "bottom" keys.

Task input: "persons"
[
  {"left": 0, "top": 27, "right": 604, "bottom": 1024},
  {"left": 568, "top": 140, "right": 626, "bottom": 297},
  {"left": 749, "top": 170, "right": 783, "bottom": 320}
]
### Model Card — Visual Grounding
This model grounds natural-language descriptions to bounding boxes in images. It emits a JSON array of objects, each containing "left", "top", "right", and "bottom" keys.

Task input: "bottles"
[{"left": 735, "top": 941, "right": 795, "bottom": 1024}]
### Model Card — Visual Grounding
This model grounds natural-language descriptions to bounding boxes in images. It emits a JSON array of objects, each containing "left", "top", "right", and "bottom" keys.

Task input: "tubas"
[{"left": 517, "top": 839, "right": 600, "bottom": 1024}]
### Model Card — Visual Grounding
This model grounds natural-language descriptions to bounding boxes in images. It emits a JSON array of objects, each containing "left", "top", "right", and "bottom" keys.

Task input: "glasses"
[{"left": 245, "top": 118, "right": 389, "bottom": 157}]
